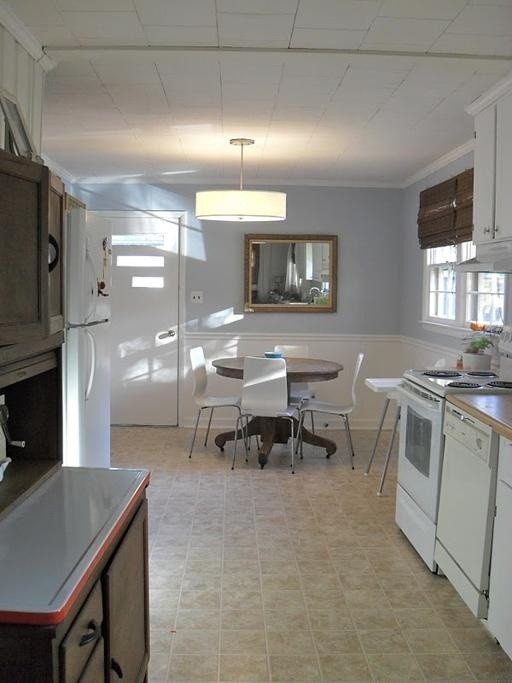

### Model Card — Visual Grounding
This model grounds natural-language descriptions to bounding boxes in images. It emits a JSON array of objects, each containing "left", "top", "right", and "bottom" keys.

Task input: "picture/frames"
[{"left": 0, "top": 89, "right": 37, "bottom": 158}]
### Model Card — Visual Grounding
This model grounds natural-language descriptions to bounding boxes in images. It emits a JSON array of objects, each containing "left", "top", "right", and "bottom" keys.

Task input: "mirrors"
[{"left": 244, "top": 233, "right": 338, "bottom": 313}]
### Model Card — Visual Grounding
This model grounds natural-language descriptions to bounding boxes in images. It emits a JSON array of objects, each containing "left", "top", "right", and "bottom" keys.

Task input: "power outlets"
[{"left": 192, "top": 291, "right": 203, "bottom": 304}]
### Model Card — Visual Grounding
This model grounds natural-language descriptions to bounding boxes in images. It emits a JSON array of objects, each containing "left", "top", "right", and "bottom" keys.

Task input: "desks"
[{"left": 212, "top": 357, "right": 343, "bottom": 469}]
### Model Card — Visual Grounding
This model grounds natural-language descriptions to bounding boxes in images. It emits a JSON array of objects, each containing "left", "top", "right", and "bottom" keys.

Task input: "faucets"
[{"left": 309, "top": 286, "right": 320, "bottom": 294}]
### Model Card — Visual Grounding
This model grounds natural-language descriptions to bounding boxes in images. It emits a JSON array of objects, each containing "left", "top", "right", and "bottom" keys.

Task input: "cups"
[{"left": 264, "top": 351, "right": 282, "bottom": 357}]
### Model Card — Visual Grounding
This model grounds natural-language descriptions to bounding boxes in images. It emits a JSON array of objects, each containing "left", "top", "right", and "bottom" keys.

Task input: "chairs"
[
  {"left": 295, "top": 352, "right": 364, "bottom": 459},
  {"left": 365, "top": 359, "right": 445, "bottom": 496},
  {"left": 189, "top": 346, "right": 251, "bottom": 462},
  {"left": 231, "top": 356, "right": 297, "bottom": 474},
  {"left": 274, "top": 345, "right": 315, "bottom": 435}
]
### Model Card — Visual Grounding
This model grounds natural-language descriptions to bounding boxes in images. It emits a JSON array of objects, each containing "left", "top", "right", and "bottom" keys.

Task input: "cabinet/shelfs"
[
  {"left": 0, "top": 150, "right": 151, "bottom": 683},
  {"left": 395, "top": 370, "right": 445, "bottom": 572},
  {"left": 434, "top": 390, "right": 512, "bottom": 661},
  {"left": 465, "top": 69, "right": 512, "bottom": 262}
]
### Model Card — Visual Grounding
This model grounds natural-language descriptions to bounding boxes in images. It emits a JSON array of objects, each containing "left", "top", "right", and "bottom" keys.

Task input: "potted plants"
[
  {"left": 471, "top": 336, "right": 494, "bottom": 370},
  {"left": 461, "top": 337, "right": 479, "bottom": 370}
]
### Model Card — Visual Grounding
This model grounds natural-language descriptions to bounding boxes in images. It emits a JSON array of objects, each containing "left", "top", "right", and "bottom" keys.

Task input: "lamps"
[{"left": 196, "top": 139, "right": 286, "bottom": 221}]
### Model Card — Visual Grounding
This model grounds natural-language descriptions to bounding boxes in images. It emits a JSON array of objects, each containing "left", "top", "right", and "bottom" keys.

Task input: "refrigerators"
[{"left": 62, "top": 206, "right": 115, "bottom": 471}]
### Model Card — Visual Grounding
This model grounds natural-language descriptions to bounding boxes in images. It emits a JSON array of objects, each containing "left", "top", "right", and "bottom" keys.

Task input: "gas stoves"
[{"left": 402, "top": 364, "right": 511, "bottom": 398}]
